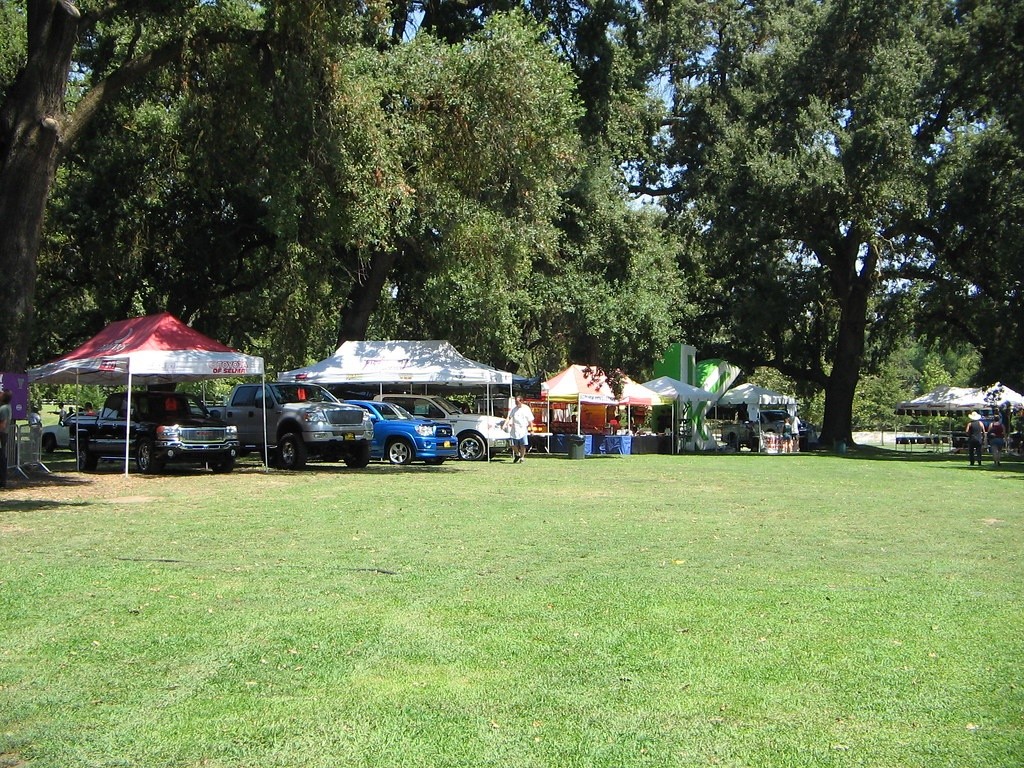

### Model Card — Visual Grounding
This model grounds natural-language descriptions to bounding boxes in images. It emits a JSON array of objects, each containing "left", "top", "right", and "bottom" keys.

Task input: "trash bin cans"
[{"left": 568, "top": 438, "right": 585, "bottom": 459}]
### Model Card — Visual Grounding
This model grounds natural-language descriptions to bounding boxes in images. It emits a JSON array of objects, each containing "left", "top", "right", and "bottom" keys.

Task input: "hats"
[{"left": 968, "top": 411, "right": 981, "bottom": 420}]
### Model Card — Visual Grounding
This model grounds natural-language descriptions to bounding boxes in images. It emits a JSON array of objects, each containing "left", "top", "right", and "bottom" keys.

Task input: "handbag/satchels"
[{"left": 990, "top": 432, "right": 996, "bottom": 437}]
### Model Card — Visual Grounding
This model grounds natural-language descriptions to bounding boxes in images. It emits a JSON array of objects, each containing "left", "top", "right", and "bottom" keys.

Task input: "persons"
[
  {"left": 657, "top": 410, "right": 666, "bottom": 434},
  {"left": 0, "top": 389, "right": 96, "bottom": 491},
  {"left": 966, "top": 412, "right": 987, "bottom": 465},
  {"left": 613, "top": 415, "right": 621, "bottom": 434},
  {"left": 502, "top": 395, "right": 534, "bottom": 463},
  {"left": 571, "top": 415, "right": 577, "bottom": 423},
  {"left": 462, "top": 406, "right": 469, "bottom": 414},
  {"left": 988, "top": 415, "right": 1006, "bottom": 467},
  {"left": 783, "top": 413, "right": 802, "bottom": 453},
  {"left": 625, "top": 420, "right": 635, "bottom": 434},
  {"left": 666, "top": 409, "right": 673, "bottom": 433}
]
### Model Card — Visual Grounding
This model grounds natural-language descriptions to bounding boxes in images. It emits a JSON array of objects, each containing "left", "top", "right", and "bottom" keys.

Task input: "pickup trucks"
[
  {"left": 189, "top": 383, "right": 375, "bottom": 471},
  {"left": 372, "top": 395, "right": 514, "bottom": 462},
  {"left": 720, "top": 409, "right": 806, "bottom": 452},
  {"left": 68, "top": 390, "right": 241, "bottom": 475}
]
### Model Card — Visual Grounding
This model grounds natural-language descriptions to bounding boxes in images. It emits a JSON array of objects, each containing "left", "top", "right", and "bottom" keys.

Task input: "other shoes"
[{"left": 513, "top": 455, "right": 524, "bottom": 463}]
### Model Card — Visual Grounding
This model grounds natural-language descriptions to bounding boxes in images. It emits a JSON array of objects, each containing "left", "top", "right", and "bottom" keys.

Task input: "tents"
[
  {"left": 714, "top": 382, "right": 796, "bottom": 453},
  {"left": 27, "top": 312, "right": 269, "bottom": 476},
  {"left": 277, "top": 340, "right": 674, "bottom": 463},
  {"left": 640, "top": 376, "right": 716, "bottom": 453},
  {"left": 894, "top": 381, "right": 1024, "bottom": 456}
]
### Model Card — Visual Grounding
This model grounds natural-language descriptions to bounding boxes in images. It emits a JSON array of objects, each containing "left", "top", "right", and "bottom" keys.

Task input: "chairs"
[{"left": 895, "top": 437, "right": 952, "bottom": 452}]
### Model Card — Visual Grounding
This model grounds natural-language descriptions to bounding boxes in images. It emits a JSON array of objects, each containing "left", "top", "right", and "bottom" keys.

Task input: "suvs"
[{"left": 341, "top": 401, "right": 459, "bottom": 465}]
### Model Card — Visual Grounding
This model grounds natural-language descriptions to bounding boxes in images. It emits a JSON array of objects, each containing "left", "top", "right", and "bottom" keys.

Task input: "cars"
[{"left": 41, "top": 411, "right": 85, "bottom": 454}]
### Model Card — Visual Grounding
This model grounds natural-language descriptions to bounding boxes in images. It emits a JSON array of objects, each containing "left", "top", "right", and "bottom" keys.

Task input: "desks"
[
  {"left": 631, "top": 436, "right": 678, "bottom": 455},
  {"left": 550, "top": 434, "right": 592, "bottom": 454},
  {"left": 593, "top": 436, "right": 631, "bottom": 455}
]
